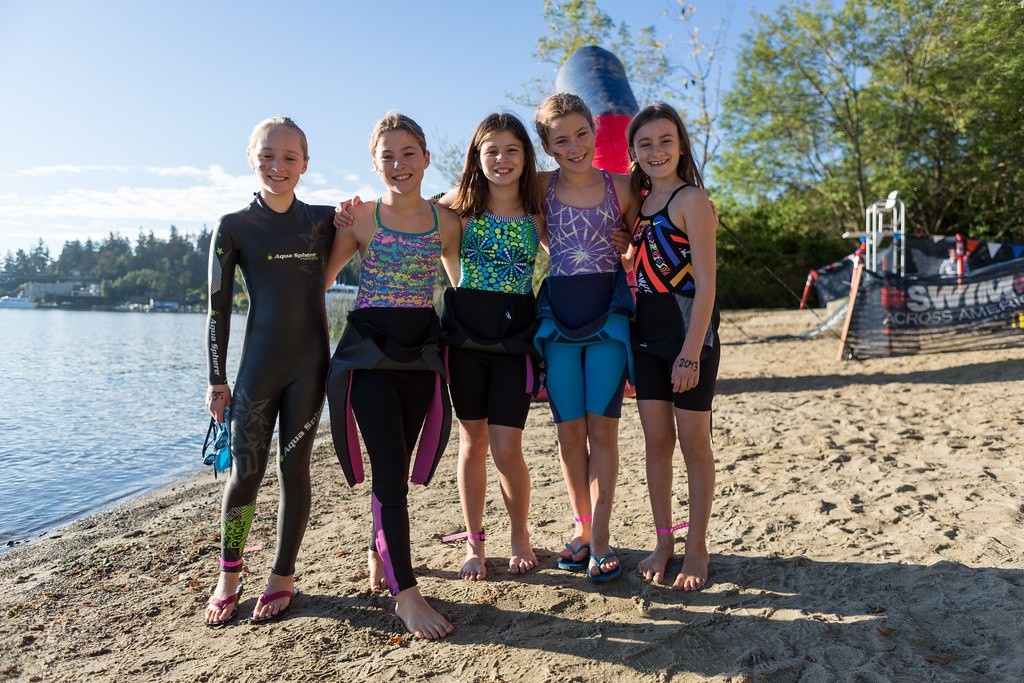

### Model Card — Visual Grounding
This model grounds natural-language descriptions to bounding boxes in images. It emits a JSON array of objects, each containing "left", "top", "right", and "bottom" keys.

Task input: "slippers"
[
  {"left": 249, "top": 587, "right": 300, "bottom": 623},
  {"left": 556, "top": 543, "right": 622, "bottom": 583},
  {"left": 203, "top": 583, "right": 243, "bottom": 625}
]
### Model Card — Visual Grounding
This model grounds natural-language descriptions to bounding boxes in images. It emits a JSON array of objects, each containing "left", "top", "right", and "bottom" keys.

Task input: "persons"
[
  {"left": 205, "top": 94, "right": 721, "bottom": 640},
  {"left": 938, "top": 248, "right": 970, "bottom": 275}
]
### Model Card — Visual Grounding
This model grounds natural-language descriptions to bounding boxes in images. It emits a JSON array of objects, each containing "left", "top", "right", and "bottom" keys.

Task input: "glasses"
[{"left": 203, "top": 416, "right": 228, "bottom": 466}]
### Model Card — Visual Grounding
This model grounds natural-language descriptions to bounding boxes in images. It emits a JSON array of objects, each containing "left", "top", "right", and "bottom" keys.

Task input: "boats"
[{"left": 0, "top": 296, "right": 39, "bottom": 308}]
